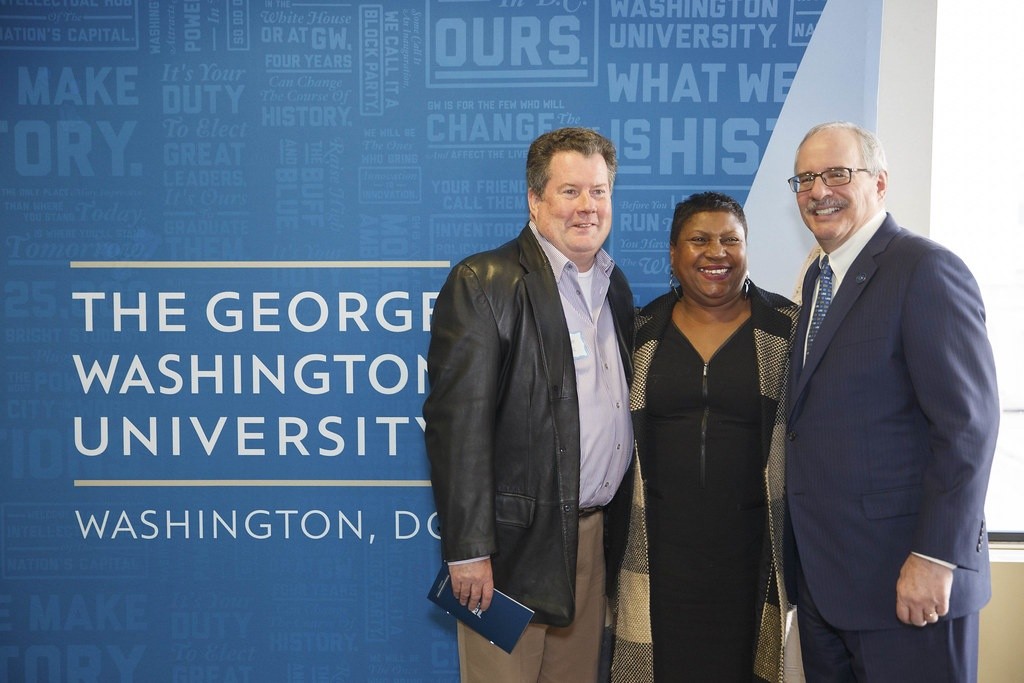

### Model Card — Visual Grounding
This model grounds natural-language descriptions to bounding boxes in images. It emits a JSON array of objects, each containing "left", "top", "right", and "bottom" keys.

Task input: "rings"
[{"left": 923, "top": 611, "right": 937, "bottom": 618}]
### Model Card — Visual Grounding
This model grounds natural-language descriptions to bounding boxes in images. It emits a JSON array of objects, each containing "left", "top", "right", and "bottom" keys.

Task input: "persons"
[
  {"left": 782, "top": 122, "right": 1000, "bottom": 683},
  {"left": 422, "top": 127, "right": 635, "bottom": 683},
  {"left": 603, "top": 191, "right": 803, "bottom": 683}
]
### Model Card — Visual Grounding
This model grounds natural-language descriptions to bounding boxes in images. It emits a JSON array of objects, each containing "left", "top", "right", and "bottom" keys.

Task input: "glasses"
[{"left": 787, "top": 166, "right": 873, "bottom": 194}]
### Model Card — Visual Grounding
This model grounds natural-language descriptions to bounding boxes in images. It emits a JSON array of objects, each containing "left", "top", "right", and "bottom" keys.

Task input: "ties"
[{"left": 806, "top": 254, "right": 833, "bottom": 365}]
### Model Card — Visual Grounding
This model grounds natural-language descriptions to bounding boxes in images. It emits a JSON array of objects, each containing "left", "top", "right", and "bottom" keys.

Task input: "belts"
[{"left": 578, "top": 505, "right": 604, "bottom": 518}]
